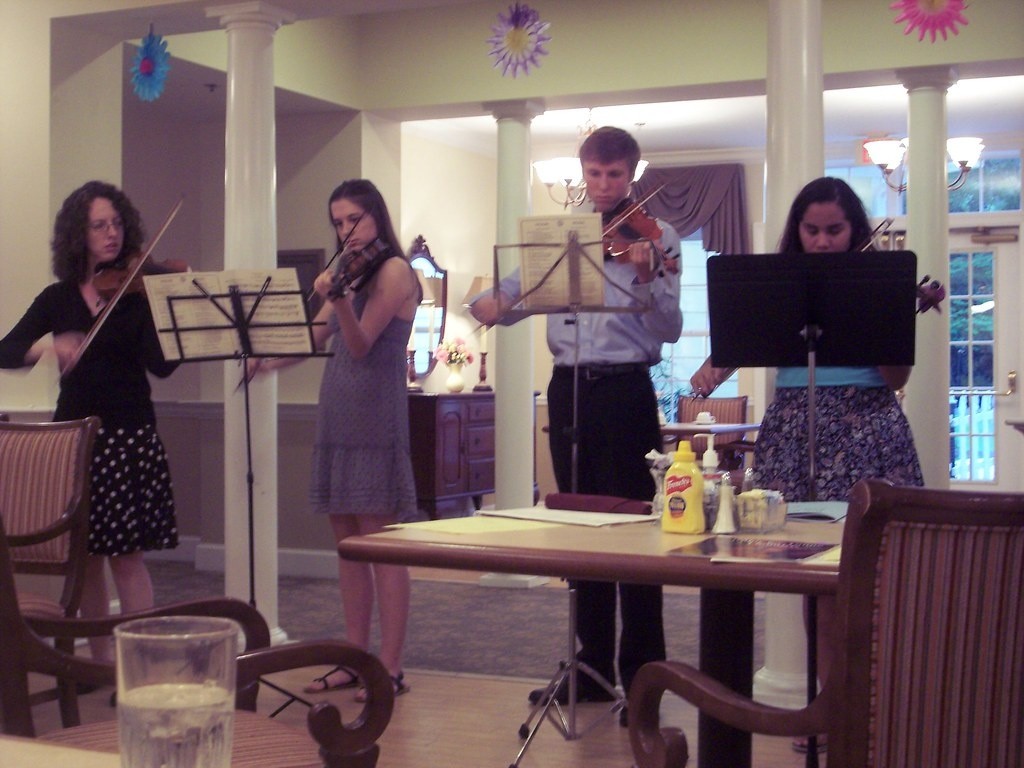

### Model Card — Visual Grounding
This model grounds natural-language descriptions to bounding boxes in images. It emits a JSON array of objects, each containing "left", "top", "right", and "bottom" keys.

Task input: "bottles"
[
  {"left": 661, "top": 441, "right": 707, "bottom": 535},
  {"left": 740, "top": 468, "right": 754, "bottom": 492},
  {"left": 712, "top": 471, "right": 736, "bottom": 533}
]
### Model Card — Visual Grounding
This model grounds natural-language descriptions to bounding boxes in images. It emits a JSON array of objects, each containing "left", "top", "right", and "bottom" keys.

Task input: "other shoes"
[
  {"left": 78, "top": 672, "right": 112, "bottom": 695},
  {"left": 529, "top": 668, "right": 615, "bottom": 706},
  {"left": 110, "top": 692, "right": 117, "bottom": 706},
  {"left": 619, "top": 706, "right": 631, "bottom": 727}
]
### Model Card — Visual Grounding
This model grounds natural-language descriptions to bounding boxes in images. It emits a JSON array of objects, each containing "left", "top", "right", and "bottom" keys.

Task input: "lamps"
[
  {"left": 862, "top": 137, "right": 985, "bottom": 193},
  {"left": 530, "top": 106, "right": 651, "bottom": 209}
]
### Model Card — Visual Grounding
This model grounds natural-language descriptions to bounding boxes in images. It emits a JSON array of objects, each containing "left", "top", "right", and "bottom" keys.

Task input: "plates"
[
  {"left": 659, "top": 423, "right": 667, "bottom": 426},
  {"left": 693, "top": 421, "right": 716, "bottom": 425}
]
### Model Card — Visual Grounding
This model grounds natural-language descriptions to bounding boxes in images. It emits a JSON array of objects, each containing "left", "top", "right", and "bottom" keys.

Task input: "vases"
[{"left": 446, "top": 364, "right": 464, "bottom": 392}]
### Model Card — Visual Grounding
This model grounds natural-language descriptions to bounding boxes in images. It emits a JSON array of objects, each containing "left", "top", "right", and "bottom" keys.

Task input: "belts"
[{"left": 553, "top": 362, "right": 650, "bottom": 380}]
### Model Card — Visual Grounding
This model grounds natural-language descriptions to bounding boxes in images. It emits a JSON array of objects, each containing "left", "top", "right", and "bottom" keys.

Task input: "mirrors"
[{"left": 405, "top": 235, "right": 447, "bottom": 380}]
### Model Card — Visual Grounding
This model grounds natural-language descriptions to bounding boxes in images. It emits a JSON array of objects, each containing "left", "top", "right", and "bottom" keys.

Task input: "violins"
[
  {"left": 325, "top": 239, "right": 392, "bottom": 302},
  {"left": 601, "top": 198, "right": 682, "bottom": 277},
  {"left": 91, "top": 249, "right": 194, "bottom": 301},
  {"left": 863, "top": 245, "right": 949, "bottom": 319}
]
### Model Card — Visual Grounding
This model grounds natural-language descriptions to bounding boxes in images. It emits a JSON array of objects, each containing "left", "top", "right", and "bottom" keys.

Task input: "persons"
[
  {"left": 0, "top": 178, "right": 192, "bottom": 708},
  {"left": 466, "top": 125, "right": 684, "bottom": 730},
  {"left": 238, "top": 180, "right": 422, "bottom": 703},
  {"left": 690, "top": 178, "right": 924, "bottom": 754}
]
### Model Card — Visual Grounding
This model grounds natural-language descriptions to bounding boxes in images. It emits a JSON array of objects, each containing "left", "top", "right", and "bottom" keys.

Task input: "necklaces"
[{"left": 94, "top": 293, "right": 103, "bottom": 308}]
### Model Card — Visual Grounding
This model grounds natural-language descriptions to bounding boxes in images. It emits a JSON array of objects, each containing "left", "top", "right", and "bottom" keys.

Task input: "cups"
[
  {"left": 696, "top": 412, "right": 715, "bottom": 422},
  {"left": 659, "top": 412, "right": 666, "bottom": 422},
  {"left": 735, "top": 503, "right": 787, "bottom": 532},
  {"left": 113, "top": 615, "right": 237, "bottom": 768}
]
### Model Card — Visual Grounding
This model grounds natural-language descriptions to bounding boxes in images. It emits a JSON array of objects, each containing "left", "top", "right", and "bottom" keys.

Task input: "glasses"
[{"left": 87, "top": 217, "right": 124, "bottom": 232}]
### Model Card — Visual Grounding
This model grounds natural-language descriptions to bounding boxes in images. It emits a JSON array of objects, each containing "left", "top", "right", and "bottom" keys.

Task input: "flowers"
[{"left": 430, "top": 337, "right": 475, "bottom": 366}]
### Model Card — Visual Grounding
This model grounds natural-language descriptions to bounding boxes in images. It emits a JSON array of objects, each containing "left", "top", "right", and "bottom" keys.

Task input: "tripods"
[{"left": 492, "top": 231, "right": 652, "bottom": 768}]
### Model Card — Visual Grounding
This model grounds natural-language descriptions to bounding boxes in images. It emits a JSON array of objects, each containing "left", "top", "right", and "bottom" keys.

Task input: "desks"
[
  {"left": 541, "top": 422, "right": 761, "bottom": 453},
  {"left": 404, "top": 389, "right": 544, "bottom": 522},
  {"left": 336, "top": 495, "right": 845, "bottom": 767}
]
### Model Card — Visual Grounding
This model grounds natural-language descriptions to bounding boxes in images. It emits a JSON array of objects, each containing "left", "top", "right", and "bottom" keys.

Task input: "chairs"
[
  {"left": 628, "top": 478, "right": 1022, "bottom": 768},
  {"left": 659, "top": 394, "right": 757, "bottom": 491},
  {"left": 1, "top": 411, "right": 102, "bottom": 729},
  {"left": 1, "top": 509, "right": 396, "bottom": 768}
]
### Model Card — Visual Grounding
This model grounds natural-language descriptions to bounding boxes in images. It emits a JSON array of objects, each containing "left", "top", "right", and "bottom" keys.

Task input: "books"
[
  {"left": 787, "top": 500, "right": 850, "bottom": 523},
  {"left": 667, "top": 537, "right": 841, "bottom": 564},
  {"left": 140, "top": 268, "right": 312, "bottom": 360},
  {"left": 519, "top": 213, "right": 604, "bottom": 311}
]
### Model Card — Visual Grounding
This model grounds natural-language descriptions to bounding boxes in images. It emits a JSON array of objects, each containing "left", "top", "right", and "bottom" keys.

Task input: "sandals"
[
  {"left": 792, "top": 742, "right": 826, "bottom": 754},
  {"left": 303, "top": 665, "right": 358, "bottom": 694},
  {"left": 355, "top": 670, "right": 410, "bottom": 703}
]
